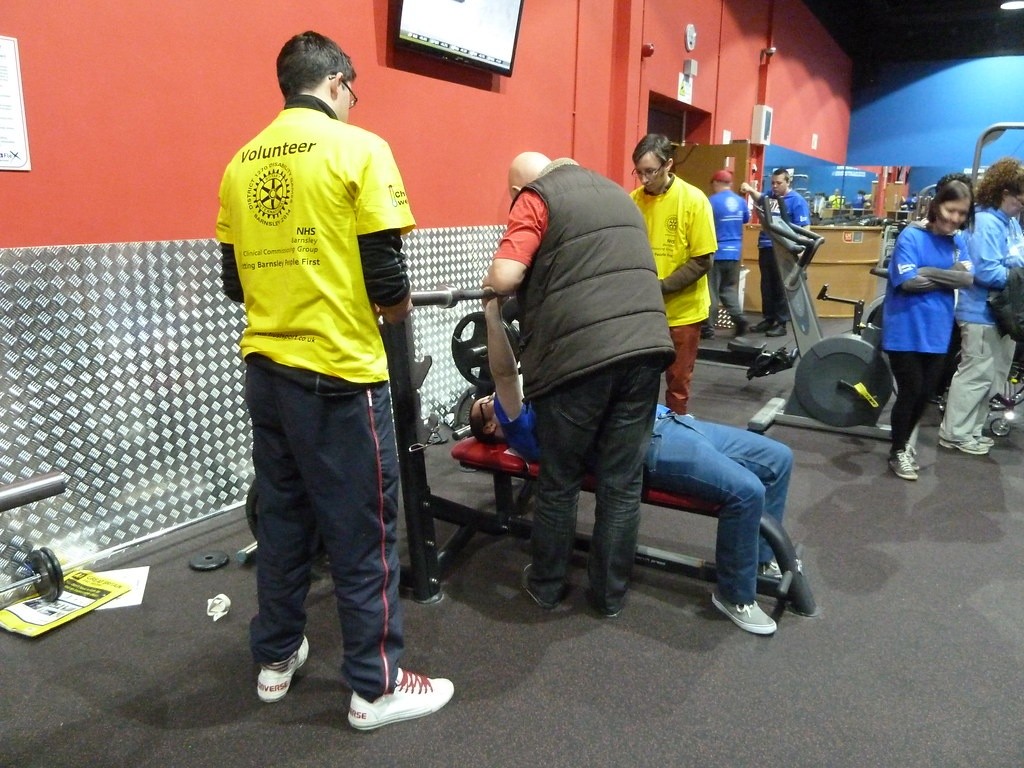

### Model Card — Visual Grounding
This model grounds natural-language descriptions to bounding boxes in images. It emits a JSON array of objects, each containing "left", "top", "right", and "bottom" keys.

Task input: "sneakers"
[
  {"left": 939, "top": 433, "right": 995, "bottom": 454},
  {"left": 888, "top": 441, "right": 921, "bottom": 481},
  {"left": 257, "top": 635, "right": 310, "bottom": 703},
  {"left": 750, "top": 320, "right": 773, "bottom": 332},
  {"left": 712, "top": 589, "right": 777, "bottom": 634},
  {"left": 765, "top": 324, "right": 788, "bottom": 336},
  {"left": 758, "top": 559, "right": 803, "bottom": 579},
  {"left": 347, "top": 668, "right": 455, "bottom": 730}
]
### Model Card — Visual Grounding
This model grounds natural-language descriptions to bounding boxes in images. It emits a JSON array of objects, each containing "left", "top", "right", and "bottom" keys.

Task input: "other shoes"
[
  {"left": 520, "top": 564, "right": 558, "bottom": 609},
  {"left": 700, "top": 328, "right": 715, "bottom": 339},
  {"left": 590, "top": 584, "right": 626, "bottom": 618},
  {"left": 733, "top": 316, "right": 748, "bottom": 336}
]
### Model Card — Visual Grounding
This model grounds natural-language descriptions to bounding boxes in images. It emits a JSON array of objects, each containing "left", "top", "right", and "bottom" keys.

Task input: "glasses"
[
  {"left": 630, "top": 163, "right": 663, "bottom": 178},
  {"left": 328, "top": 75, "right": 358, "bottom": 109}
]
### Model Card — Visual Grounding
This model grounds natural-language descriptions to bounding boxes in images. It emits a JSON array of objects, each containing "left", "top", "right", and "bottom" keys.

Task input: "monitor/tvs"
[{"left": 394, "top": 0, "right": 524, "bottom": 78}]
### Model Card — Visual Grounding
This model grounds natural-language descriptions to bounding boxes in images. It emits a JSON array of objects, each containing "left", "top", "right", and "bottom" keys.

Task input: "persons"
[
  {"left": 216, "top": 31, "right": 456, "bottom": 732},
  {"left": 898, "top": 192, "right": 918, "bottom": 219},
  {"left": 740, "top": 168, "right": 811, "bottom": 336},
  {"left": 813, "top": 189, "right": 871, "bottom": 218},
  {"left": 938, "top": 157, "right": 1024, "bottom": 454},
  {"left": 483, "top": 152, "right": 677, "bottom": 617},
  {"left": 470, "top": 285, "right": 795, "bottom": 634},
  {"left": 628, "top": 134, "right": 721, "bottom": 417},
  {"left": 881, "top": 180, "right": 976, "bottom": 478},
  {"left": 702, "top": 170, "right": 750, "bottom": 338}
]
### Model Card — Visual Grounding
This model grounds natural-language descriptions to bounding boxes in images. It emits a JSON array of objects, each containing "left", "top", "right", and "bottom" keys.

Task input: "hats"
[{"left": 710, "top": 171, "right": 734, "bottom": 184}]
